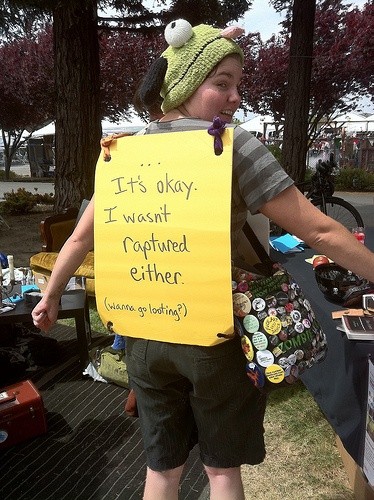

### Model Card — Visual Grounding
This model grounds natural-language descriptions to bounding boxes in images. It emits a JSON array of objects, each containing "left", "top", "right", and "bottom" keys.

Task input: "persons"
[{"left": 31, "top": 18, "right": 374, "bottom": 500}]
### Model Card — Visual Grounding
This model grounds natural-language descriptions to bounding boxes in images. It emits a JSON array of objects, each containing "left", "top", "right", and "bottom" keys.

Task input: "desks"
[
  {"left": 0, "top": 285, "right": 90, "bottom": 359},
  {"left": 268, "top": 228, "right": 374, "bottom": 500}
]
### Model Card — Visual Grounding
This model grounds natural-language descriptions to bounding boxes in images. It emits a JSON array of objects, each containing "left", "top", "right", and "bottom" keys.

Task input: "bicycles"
[{"left": 267, "top": 159, "right": 364, "bottom": 249}]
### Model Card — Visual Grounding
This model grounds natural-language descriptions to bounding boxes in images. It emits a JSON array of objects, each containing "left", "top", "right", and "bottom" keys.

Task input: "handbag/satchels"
[{"left": 232, "top": 261, "right": 329, "bottom": 391}]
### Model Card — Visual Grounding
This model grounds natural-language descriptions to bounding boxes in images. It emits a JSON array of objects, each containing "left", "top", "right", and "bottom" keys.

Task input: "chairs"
[{"left": 29, "top": 204, "right": 94, "bottom": 298}]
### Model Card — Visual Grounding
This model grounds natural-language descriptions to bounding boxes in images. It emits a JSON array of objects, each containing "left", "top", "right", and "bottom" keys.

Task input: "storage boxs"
[{"left": 0, "top": 379, "right": 47, "bottom": 449}]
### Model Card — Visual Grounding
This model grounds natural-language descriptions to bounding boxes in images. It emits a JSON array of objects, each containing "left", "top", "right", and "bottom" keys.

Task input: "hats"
[{"left": 134, "top": 18, "right": 244, "bottom": 115}]
